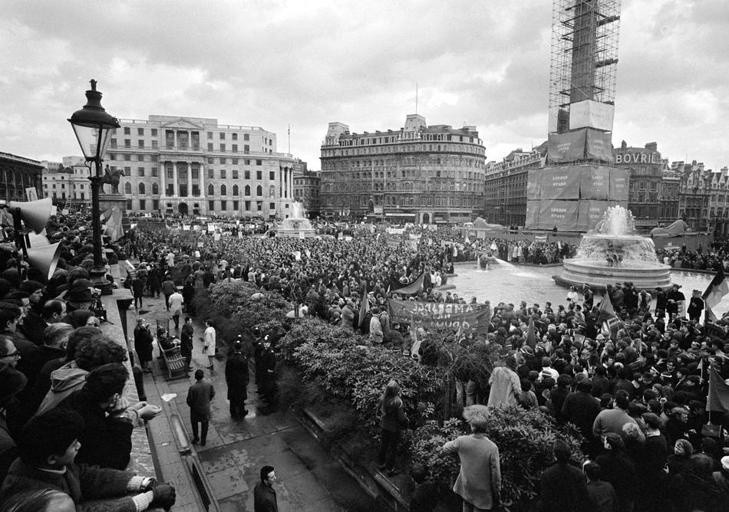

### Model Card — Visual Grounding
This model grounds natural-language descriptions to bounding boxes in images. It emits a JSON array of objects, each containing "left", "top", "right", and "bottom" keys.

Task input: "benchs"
[{"left": 156, "top": 336, "right": 191, "bottom": 379}]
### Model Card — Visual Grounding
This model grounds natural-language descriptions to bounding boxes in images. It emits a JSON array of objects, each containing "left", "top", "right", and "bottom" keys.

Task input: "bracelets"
[{"left": 130, "top": 406, "right": 142, "bottom": 423}]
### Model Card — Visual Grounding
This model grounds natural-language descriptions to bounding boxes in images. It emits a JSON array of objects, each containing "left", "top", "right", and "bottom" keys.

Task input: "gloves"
[{"left": 147, "top": 481, "right": 180, "bottom": 508}]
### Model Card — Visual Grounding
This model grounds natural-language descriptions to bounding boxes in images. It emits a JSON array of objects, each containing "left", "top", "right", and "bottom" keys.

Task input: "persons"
[
  {"left": 309, "top": 211, "right": 453, "bottom": 283},
  {"left": 47, "top": 267, "right": 108, "bottom": 328},
  {"left": 0, "top": 267, "right": 46, "bottom": 451},
  {"left": 189, "top": 237, "right": 308, "bottom": 305},
  {"left": 253, "top": 465, "right": 279, "bottom": 512},
  {"left": 186, "top": 370, "right": 215, "bottom": 447},
  {"left": 224, "top": 341, "right": 249, "bottom": 422},
  {"left": 25, "top": 328, "right": 128, "bottom": 424},
  {"left": 199, "top": 319, "right": 217, "bottom": 373},
  {"left": 134, "top": 320, "right": 154, "bottom": 374},
  {"left": 122, "top": 208, "right": 283, "bottom": 236},
  {"left": 56, "top": 362, "right": 162, "bottom": 471},
  {"left": 0, "top": 427, "right": 176, "bottom": 511},
  {"left": 437, "top": 402, "right": 501, "bottom": 511},
  {"left": 452, "top": 214, "right": 576, "bottom": 270},
  {"left": 655, "top": 244, "right": 727, "bottom": 274},
  {"left": 306, "top": 280, "right": 487, "bottom": 407},
  {"left": 254, "top": 334, "right": 276, "bottom": 404},
  {"left": 125, "top": 238, "right": 191, "bottom": 332},
  {"left": 180, "top": 316, "right": 195, "bottom": 374},
  {"left": 0, "top": 198, "right": 105, "bottom": 267}
]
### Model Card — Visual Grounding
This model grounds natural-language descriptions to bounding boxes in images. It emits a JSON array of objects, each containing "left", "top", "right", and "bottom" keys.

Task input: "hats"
[{"left": 62, "top": 283, "right": 93, "bottom": 306}]
